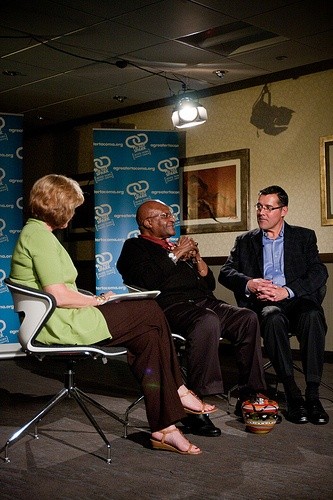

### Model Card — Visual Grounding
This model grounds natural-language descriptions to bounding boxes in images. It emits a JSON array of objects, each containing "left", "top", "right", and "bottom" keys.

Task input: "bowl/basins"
[{"left": 241, "top": 397, "right": 279, "bottom": 434}]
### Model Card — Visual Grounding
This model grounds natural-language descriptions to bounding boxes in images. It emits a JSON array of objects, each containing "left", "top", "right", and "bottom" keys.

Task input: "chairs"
[
  {"left": 233, "top": 264, "right": 333, "bottom": 402},
  {"left": 0, "top": 277, "right": 127, "bottom": 465},
  {"left": 122, "top": 281, "right": 223, "bottom": 421}
]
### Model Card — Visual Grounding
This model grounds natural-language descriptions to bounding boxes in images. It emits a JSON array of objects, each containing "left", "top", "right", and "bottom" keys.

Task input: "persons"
[
  {"left": 217, "top": 186, "right": 331, "bottom": 426},
  {"left": 117, "top": 201, "right": 283, "bottom": 426},
  {"left": 10, "top": 174, "right": 217, "bottom": 454}
]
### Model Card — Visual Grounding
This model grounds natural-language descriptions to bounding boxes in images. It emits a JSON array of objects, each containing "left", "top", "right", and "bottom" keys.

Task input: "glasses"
[
  {"left": 145, "top": 213, "right": 175, "bottom": 220},
  {"left": 254, "top": 203, "right": 285, "bottom": 212}
]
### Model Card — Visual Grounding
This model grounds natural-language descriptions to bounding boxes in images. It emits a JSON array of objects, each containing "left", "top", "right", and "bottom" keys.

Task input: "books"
[{"left": 108, "top": 290, "right": 161, "bottom": 301}]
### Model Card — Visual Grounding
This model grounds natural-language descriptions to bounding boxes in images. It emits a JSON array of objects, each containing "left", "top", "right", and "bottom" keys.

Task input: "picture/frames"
[
  {"left": 319, "top": 135, "right": 333, "bottom": 226},
  {"left": 180, "top": 148, "right": 250, "bottom": 235}
]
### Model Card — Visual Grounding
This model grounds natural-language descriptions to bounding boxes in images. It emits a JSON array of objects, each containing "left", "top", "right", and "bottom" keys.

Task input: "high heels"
[
  {"left": 150, "top": 429, "right": 202, "bottom": 454},
  {"left": 178, "top": 390, "right": 217, "bottom": 414}
]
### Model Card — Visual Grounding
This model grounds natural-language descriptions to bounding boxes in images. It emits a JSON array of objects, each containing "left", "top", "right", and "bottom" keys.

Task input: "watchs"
[{"left": 167, "top": 251, "right": 178, "bottom": 264}]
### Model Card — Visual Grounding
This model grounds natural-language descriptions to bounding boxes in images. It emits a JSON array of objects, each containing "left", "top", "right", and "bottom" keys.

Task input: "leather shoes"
[
  {"left": 288, "top": 400, "right": 308, "bottom": 424},
  {"left": 304, "top": 398, "right": 330, "bottom": 424},
  {"left": 235, "top": 398, "right": 282, "bottom": 424},
  {"left": 179, "top": 414, "right": 220, "bottom": 437}
]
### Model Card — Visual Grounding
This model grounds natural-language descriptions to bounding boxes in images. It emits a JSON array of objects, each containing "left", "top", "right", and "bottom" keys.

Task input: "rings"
[{"left": 274, "top": 296, "right": 277, "bottom": 301}]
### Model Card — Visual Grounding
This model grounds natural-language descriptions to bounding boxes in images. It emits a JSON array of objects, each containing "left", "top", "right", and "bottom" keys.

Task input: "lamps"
[{"left": 171, "top": 83, "right": 208, "bottom": 129}]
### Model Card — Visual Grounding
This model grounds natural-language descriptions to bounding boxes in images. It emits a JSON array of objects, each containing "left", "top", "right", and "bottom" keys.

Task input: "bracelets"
[{"left": 95, "top": 296, "right": 106, "bottom": 307}]
